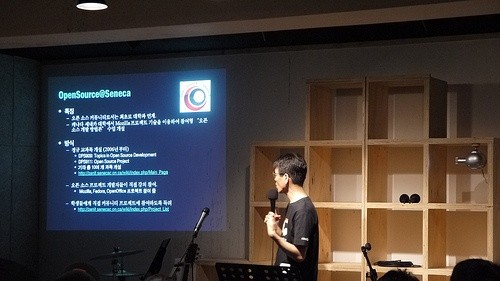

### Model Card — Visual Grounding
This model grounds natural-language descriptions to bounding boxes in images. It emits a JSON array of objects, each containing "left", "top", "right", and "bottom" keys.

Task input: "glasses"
[{"left": 272, "top": 171, "right": 280, "bottom": 177}]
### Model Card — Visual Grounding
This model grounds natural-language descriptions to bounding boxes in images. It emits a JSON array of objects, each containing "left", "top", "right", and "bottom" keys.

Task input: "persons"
[
  {"left": 56, "top": 271, "right": 96, "bottom": 281},
  {"left": 263, "top": 152, "right": 318, "bottom": 281},
  {"left": 450, "top": 258, "right": 500, "bottom": 281},
  {"left": 377, "top": 269, "right": 420, "bottom": 281}
]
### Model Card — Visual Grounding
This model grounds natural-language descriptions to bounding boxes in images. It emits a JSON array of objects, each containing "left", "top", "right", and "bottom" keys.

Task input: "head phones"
[{"left": 400, "top": 193, "right": 421, "bottom": 203}]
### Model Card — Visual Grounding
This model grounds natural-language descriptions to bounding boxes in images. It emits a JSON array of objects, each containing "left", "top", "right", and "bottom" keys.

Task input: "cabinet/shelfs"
[{"left": 196, "top": 76, "right": 499, "bottom": 281}]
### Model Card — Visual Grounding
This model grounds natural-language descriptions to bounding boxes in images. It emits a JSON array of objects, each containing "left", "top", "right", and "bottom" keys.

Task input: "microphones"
[
  {"left": 267, "top": 188, "right": 278, "bottom": 214},
  {"left": 194, "top": 207, "right": 209, "bottom": 235}
]
